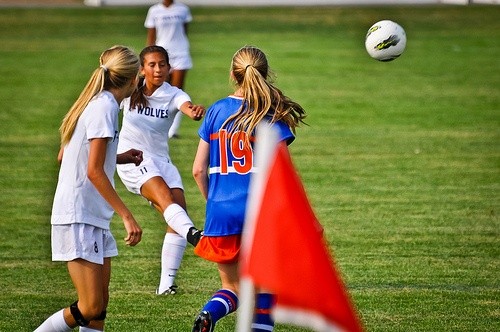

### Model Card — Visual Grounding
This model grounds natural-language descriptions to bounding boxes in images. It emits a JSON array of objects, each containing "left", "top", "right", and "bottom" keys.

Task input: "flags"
[{"left": 237, "top": 124, "right": 366, "bottom": 332}]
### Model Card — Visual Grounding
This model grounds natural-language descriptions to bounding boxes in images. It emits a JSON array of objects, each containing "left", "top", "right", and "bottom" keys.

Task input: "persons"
[
  {"left": 143, "top": 0, "right": 193, "bottom": 137},
  {"left": 116, "top": 45, "right": 206, "bottom": 295},
  {"left": 32, "top": 44, "right": 143, "bottom": 332},
  {"left": 192, "top": 46, "right": 312, "bottom": 332}
]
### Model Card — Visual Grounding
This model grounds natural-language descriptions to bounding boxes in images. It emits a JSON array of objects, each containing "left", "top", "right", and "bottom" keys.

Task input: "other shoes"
[
  {"left": 155, "top": 287, "right": 177, "bottom": 296},
  {"left": 187, "top": 227, "right": 203, "bottom": 247},
  {"left": 192, "top": 312, "right": 214, "bottom": 332}
]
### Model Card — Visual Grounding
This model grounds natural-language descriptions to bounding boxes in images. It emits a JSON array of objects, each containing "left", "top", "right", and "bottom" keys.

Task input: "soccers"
[{"left": 364, "top": 19, "right": 408, "bottom": 63}]
